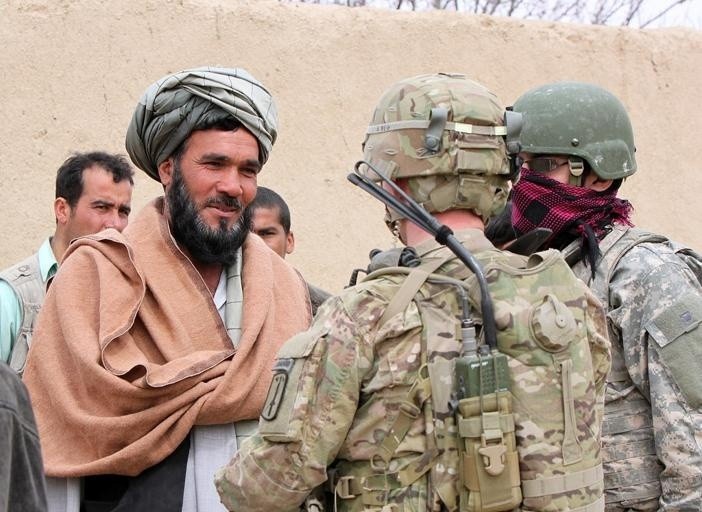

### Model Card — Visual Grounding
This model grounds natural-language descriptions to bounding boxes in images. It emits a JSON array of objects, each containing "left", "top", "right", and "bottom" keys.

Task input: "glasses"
[{"left": 514, "top": 155, "right": 568, "bottom": 173}]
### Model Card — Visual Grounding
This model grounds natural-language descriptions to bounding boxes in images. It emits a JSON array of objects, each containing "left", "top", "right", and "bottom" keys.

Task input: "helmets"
[
  {"left": 364, "top": 73, "right": 510, "bottom": 219},
  {"left": 514, "top": 83, "right": 637, "bottom": 181}
]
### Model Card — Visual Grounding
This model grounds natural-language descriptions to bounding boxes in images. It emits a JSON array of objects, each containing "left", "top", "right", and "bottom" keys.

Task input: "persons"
[
  {"left": 489, "top": 82, "right": 702, "bottom": 512},
  {"left": 214, "top": 74, "right": 613, "bottom": 512},
  {"left": 0, "top": 364, "right": 49, "bottom": 512},
  {"left": 243, "top": 186, "right": 334, "bottom": 314},
  {"left": 21, "top": 68, "right": 312, "bottom": 511},
  {"left": 1, "top": 151, "right": 133, "bottom": 366}
]
